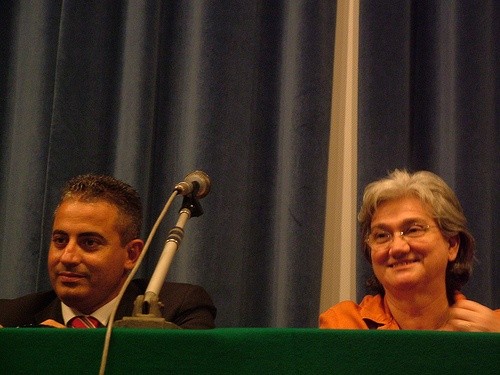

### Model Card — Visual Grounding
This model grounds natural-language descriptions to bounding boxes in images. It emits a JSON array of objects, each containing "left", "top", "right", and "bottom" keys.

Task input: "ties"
[{"left": 69, "top": 316, "right": 98, "bottom": 329}]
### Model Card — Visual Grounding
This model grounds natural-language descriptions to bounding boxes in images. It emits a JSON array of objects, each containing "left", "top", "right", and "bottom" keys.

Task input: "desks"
[{"left": 0, "top": 327, "right": 500, "bottom": 375}]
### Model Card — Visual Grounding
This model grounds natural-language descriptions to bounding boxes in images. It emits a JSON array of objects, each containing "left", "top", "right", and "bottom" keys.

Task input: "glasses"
[{"left": 364, "top": 221, "right": 440, "bottom": 247}]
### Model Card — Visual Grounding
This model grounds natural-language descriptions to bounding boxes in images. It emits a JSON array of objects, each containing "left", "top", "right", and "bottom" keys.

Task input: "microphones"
[{"left": 172, "top": 170, "right": 212, "bottom": 199}]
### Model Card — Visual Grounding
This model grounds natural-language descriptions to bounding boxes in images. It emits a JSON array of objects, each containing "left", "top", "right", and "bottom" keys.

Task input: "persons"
[
  {"left": 0, "top": 172, "right": 218, "bottom": 330},
  {"left": 318, "top": 169, "right": 500, "bottom": 332}
]
